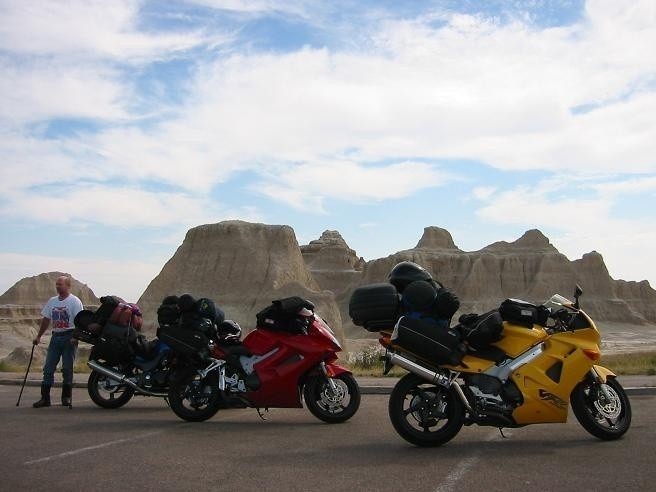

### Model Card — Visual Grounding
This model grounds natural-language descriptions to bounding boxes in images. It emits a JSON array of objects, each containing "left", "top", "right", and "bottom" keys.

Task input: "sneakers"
[
  {"left": 31, "top": 399, "right": 51, "bottom": 409},
  {"left": 60, "top": 396, "right": 71, "bottom": 407}
]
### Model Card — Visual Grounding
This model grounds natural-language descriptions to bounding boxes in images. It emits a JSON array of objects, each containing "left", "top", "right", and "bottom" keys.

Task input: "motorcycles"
[
  {"left": 349, "top": 283, "right": 632, "bottom": 447},
  {"left": 71, "top": 307, "right": 361, "bottom": 423}
]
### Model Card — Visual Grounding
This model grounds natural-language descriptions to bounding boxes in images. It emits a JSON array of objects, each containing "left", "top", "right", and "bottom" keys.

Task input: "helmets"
[
  {"left": 385, "top": 260, "right": 433, "bottom": 295},
  {"left": 214, "top": 319, "right": 242, "bottom": 345}
]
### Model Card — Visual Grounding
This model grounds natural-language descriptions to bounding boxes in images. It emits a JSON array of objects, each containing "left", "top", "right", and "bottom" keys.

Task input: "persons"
[{"left": 31, "top": 275, "right": 85, "bottom": 409}]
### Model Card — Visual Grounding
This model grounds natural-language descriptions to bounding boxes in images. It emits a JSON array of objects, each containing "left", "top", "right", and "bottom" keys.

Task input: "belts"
[{"left": 50, "top": 329, "right": 74, "bottom": 337}]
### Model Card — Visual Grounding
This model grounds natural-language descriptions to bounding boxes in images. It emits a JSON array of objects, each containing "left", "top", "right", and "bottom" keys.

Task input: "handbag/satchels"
[
  {"left": 194, "top": 297, "right": 225, "bottom": 325},
  {"left": 93, "top": 295, "right": 143, "bottom": 331},
  {"left": 432, "top": 279, "right": 461, "bottom": 320}
]
